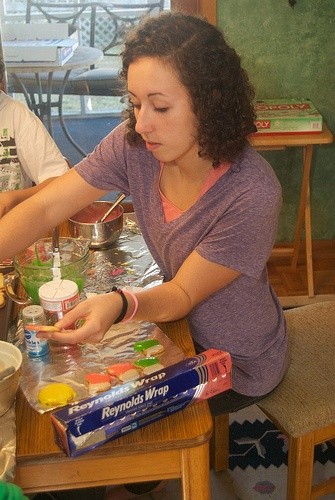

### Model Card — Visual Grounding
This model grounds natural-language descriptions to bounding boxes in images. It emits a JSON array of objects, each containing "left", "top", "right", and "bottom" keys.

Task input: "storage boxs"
[
  {"left": 2, "top": 23, "right": 79, "bottom": 67},
  {"left": 50, "top": 348, "right": 232, "bottom": 458},
  {"left": 250, "top": 99, "right": 322, "bottom": 137}
]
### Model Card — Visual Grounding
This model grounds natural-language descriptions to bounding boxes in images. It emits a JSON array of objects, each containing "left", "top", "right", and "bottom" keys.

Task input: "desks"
[
  {"left": 5, "top": 45, "right": 104, "bottom": 156},
  {"left": 246, "top": 120, "right": 333, "bottom": 299},
  {"left": 13, "top": 212, "right": 214, "bottom": 500}
]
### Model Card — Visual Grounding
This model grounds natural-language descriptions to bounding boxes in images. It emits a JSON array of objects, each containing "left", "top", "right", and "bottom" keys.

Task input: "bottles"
[
  {"left": 38, "top": 279, "right": 81, "bottom": 347},
  {"left": 21, "top": 305, "right": 49, "bottom": 357}
]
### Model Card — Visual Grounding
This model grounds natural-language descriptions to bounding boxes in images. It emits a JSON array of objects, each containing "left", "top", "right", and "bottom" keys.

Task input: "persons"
[
  {"left": 0, "top": 89, "right": 70, "bottom": 219},
  {"left": 0, "top": 11, "right": 287, "bottom": 500}
]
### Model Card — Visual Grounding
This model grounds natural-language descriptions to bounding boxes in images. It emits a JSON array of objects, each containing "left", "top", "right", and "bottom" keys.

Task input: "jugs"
[{"left": 2, "top": 238, "right": 91, "bottom": 305}]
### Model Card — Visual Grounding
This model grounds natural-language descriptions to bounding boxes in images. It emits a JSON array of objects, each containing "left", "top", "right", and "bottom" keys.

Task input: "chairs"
[{"left": 209, "top": 301, "right": 335, "bottom": 500}]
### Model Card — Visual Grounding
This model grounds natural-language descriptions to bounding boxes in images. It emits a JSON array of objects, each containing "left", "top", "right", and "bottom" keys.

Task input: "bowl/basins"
[
  {"left": 66, "top": 201, "right": 124, "bottom": 248},
  {"left": 0, "top": 340, "right": 23, "bottom": 417}
]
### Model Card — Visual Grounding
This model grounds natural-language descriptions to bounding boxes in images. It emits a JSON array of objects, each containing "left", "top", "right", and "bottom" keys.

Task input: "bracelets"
[
  {"left": 105, "top": 285, "right": 127, "bottom": 324},
  {"left": 119, "top": 290, "right": 139, "bottom": 325}
]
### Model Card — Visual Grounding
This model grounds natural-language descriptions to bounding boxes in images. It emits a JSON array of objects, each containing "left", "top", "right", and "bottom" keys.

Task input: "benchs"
[{"left": 7, "top": 0, "right": 165, "bottom": 121}]
